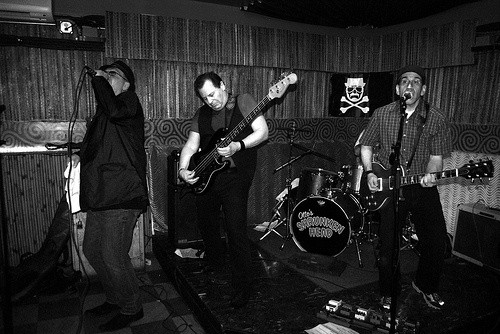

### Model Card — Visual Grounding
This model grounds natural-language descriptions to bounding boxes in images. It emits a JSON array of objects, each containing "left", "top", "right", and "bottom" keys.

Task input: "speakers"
[
  {"left": 451, "top": 202, "right": 500, "bottom": 270},
  {"left": 167, "top": 149, "right": 225, "bottom": 247}
]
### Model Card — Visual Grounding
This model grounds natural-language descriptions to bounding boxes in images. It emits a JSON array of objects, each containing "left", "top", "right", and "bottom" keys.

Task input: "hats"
[
  {"left": 397, "top": 64, "right": 427, "bottom": 83},
  {"left": 97, "top": 61, "right": 135, "bottom": 87}
]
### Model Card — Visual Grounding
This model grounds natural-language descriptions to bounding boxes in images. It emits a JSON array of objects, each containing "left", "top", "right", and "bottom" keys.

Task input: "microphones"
[
  {"left": 400, "top": 91, "right": 412, "bottom": 102},
  {"left": 85, "top": 65, "right": 96, "bottom": 77}
]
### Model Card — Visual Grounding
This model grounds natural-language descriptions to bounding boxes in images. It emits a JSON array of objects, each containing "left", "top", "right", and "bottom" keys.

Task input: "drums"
[
  {"left": 295, "top": 167, "right": 343, "bottom": 201},
  {"left": 289, "top": 188, "right": 364, "bottom": 258},
  {"left": 345, "top": 164, "right": 364, "bottom": 195}
]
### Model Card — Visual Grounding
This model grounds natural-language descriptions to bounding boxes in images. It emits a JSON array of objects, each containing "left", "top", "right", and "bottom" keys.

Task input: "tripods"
[{"left": 260, "top": 121, "right": 310, "bottom": 251}]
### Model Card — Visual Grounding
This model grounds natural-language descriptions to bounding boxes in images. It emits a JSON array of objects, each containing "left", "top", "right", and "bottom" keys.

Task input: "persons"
[
  {"left": 179, "top": 72, "right": 269, "bottom": 308},
  {"left": 67, "top": 61, "right": 150, "bottom": 331},
  {"left": 360, "top": 65, "right": 453, "bottom": 314}
]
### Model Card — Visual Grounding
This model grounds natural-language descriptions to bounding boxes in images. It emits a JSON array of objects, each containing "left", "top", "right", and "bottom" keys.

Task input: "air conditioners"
[{"left": 0, "top": 0, "right": 57, "bottom": 26}]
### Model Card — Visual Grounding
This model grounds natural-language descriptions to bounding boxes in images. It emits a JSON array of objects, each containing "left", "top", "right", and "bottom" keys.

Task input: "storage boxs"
[{"left": 451, "top": 204, "right": 500, "bottom": 275}]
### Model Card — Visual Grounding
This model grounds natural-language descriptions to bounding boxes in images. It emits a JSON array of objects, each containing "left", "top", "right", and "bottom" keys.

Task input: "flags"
[{"left": 329, "top": 72, "right": 393, "bottom": 117}]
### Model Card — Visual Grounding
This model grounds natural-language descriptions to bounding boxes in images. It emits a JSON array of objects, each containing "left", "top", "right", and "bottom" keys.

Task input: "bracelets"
[
  {"left": 178, "top": 168, "right": 185, "bottom": 178},
  {"left": 365, "top": 170, "right": 373, "bottom": 174},
  {"left": 239, "top": 140, "right": 245, "bottom": 152}
]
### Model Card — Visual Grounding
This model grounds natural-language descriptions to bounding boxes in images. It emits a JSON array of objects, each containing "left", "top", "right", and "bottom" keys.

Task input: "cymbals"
[
  {"left": 275, "top": 126, "right": 311, "bottom": 132},
  {"left": 291, "top": 142, "right": 336, "bottom": 162}
]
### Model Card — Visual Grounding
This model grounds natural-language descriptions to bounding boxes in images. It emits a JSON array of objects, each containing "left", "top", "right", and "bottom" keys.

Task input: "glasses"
[
  {"left": 103, "top": 69, "right": 128, "bottom": 82},
  {"left": 399, "top": 77, "right": 423, "bottom": 86}
]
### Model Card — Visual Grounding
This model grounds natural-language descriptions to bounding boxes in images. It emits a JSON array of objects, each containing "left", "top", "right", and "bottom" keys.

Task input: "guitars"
[
  {"left": 359, "top": 158, "right": 494, "bottom": 212},
  {"left": 187, "top": 71, "right": 298, "bottom": 194}
]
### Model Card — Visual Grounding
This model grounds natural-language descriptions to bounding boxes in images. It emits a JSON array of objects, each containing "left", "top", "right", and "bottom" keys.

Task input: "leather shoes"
[
  {"left": 85, "top": 302, "right": 121, "bottom": 317},
  {"left": 100, "top": 306, "right": 144, "bottom": 331}
]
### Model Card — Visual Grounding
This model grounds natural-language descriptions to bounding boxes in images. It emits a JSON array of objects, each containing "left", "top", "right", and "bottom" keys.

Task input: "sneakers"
[
  {"left": 411, "top": 280, "right": 448, "bottom": 310},
  {"left": 382, "top": 296, "right": 392, "bottom": 309}
]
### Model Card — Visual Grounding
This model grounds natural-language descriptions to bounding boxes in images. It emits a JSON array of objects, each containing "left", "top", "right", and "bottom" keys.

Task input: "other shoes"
[{"left": 232, "top": 285, "right": 248, "bottom": 306}]
